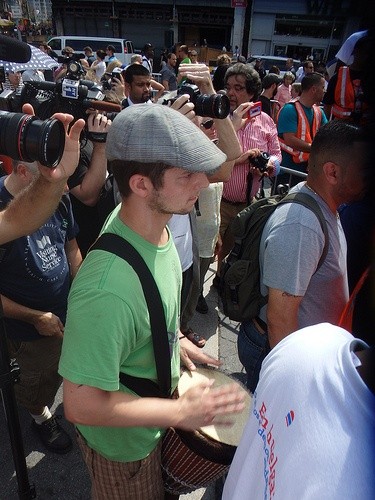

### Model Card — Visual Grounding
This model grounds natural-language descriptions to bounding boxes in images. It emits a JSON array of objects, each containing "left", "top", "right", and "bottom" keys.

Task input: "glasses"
[{"left": 224, "top": 86, "right": 246, "bottom": 92}]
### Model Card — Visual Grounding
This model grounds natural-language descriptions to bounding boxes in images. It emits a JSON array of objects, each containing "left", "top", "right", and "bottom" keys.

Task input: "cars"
[{"left": 246, "top": 55, "right": 301, "bottom": 80}]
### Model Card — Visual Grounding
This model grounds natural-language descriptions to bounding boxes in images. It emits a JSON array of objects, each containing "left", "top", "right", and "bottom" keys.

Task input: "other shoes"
[
  {"left": 182, "top": 328, "right": 207, "bottom": 347},
  {"left": 196, "top": 290, "right": 209, "bottom": 314},
  {"left": 30, "top": 414, "right": 74, "bottom": 454},
  {"left": 9, "top": 352, "right": 22, "bottom": 384}
]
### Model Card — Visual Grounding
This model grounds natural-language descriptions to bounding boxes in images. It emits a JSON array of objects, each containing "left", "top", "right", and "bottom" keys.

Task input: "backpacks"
[{"left": 215, "top": 183, "right": 329, "bottom": 322}]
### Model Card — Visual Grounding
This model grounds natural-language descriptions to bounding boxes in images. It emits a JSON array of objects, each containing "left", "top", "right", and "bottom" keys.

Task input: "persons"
[{"left": 0, "top": 13, "right": 375, "bottom": 500}]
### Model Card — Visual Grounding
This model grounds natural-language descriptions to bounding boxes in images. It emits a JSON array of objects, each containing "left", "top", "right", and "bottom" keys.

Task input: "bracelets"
[{"left": 179, "top": 336, "right": 186, "bottom": 340}]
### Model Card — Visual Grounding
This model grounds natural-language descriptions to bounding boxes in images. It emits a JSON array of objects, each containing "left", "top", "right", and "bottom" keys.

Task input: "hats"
[
  {"left": 61, "top": 47, "right": 76, "bottom": 55},
  {"left": 104, "top": 100, "right": 227, "bottom": 173},
  {"left": 256, "top": 57, "right": 262, "bottom": 63}
]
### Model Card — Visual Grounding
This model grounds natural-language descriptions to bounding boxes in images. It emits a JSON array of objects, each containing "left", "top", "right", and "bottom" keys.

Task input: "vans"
[{"left": 46, "top": 35, "right": 153, "bottom": 70}]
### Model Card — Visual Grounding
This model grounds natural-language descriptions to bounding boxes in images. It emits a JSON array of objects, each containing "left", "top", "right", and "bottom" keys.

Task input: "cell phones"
[{"left": 112, "top": 72, "right": 120, "bottom": 83}]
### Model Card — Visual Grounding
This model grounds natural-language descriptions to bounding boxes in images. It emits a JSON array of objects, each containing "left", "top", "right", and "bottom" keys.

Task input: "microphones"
[
  {"left": 82, "top": 99, "right": 121, "bottom": 112},
  {"left": 28, "top": 79, "right": 88, "bottom": 98},
  {"left": 0, "top": 34, "right": 32, "bottom": 64}
]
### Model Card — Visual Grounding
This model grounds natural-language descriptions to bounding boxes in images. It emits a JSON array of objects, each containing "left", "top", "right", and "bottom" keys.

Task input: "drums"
[{"left": 161, "top": 364, "right": 254, "bottom": 495}]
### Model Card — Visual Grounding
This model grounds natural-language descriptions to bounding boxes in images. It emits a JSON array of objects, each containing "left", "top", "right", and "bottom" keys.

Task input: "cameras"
[
  {"left": 163, "top": 83, "right": 230, "bottom": 118},
  {"left": 249, "top": 151, "right": 270, "bottom": 172}
]
